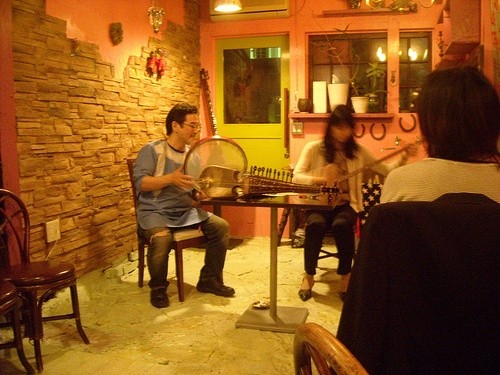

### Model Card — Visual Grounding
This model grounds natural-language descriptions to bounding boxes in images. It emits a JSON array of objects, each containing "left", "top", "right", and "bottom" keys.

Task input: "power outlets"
[{"left": 45, "top": 219, "right": 61, "bottom": 243}]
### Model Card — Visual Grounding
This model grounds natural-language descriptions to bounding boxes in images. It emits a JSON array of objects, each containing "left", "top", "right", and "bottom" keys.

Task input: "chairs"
[
  {"left": 289, "top": 207, "right": 359, "bottom": 260},
  {"left": 126, "top": 159, "right": 223, "bottom": 302},
  {"left": 0, "top": 188, "right": 89, "bottom": 375}
]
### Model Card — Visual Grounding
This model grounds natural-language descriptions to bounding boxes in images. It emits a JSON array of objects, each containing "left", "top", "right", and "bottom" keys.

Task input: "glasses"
[{"left": 178, "top": 122, "right": 200, "bottom": 130}]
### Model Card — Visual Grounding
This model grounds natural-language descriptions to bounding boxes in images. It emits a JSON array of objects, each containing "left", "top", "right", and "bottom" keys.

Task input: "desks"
[{"left": 201, "top": 194, "right": 340, "bottom": 334}]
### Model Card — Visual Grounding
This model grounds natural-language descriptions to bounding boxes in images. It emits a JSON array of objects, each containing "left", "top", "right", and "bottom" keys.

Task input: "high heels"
[
  {"left": 340, "top": 282, "right": 349, "bottom": 301},
  {"left": 298, "top": 277, "right": 315, "bottom": 301}
]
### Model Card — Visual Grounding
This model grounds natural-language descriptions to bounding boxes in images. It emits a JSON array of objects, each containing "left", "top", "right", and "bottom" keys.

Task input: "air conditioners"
[{"left": 209, "top": 0, "right": 290, "bottom": 22}]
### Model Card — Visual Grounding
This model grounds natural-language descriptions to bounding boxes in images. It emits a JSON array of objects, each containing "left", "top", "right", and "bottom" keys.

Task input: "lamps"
[{"left": 214, "top": 0, "right": 242, "bottom": 12}]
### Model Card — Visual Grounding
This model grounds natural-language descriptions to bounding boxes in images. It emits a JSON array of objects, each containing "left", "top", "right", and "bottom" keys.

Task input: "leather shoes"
[
  {"left": 196, "top": 280, "right": 235, "bottom": 297},
  {"left": 149, "top": 288, "right": 168, "bottom": 308}
]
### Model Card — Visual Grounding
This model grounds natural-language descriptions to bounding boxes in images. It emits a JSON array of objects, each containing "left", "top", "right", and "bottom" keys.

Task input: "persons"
[
  {"left": 133, "top": 102, "right": 235, "bottom": 308},
  {"left": 292, "top": 105, "right": 419, "bottom": 301},
  {"left": 380, "top": 66, "right": 500, "bottom": 203}
]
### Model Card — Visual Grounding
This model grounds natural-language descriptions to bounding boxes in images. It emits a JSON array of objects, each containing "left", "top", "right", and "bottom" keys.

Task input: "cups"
[
  {"left": 327, "top": 84, "right": 348, "bottom": 112},
  {"left": 297, "top": 99, "right": 311, "bottom": 113},
  {"left": 350, "top": 96, "right": 369, "bottom": 113}
]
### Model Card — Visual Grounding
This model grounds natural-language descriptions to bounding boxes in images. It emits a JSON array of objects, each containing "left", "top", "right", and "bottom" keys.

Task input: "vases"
[
  {"left": 351, "top": 97, "right": 369, "bottom": 113},
  {"left": 327, "top": 84, "right": 348, "bottom": 113}
]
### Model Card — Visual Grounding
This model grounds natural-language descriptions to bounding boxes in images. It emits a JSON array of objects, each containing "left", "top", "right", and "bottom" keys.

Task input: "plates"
[{"left": 252, "top": 301, "right": 270, "bottom": 309}]
